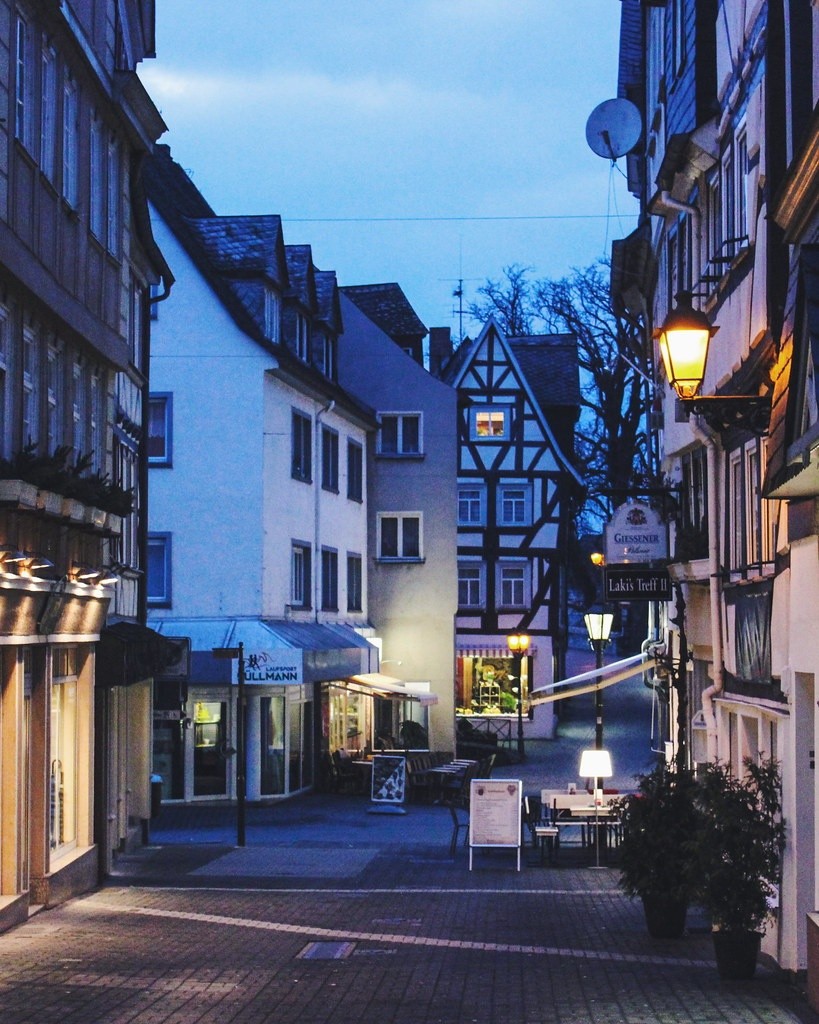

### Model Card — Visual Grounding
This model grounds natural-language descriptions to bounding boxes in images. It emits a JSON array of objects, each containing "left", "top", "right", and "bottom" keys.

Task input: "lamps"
[
  {"left": 580, "top": 750, "right": 613, "bottom": 871},
  {"left": 0, "top": 544, "right": 120, "bottom": 585},
  {"left": 653, "top": 289, "right": 773, "bottom": 436}
]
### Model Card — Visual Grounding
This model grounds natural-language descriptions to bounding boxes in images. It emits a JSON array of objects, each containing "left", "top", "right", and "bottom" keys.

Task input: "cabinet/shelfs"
[{"left": 480, "top": 686, "right": 500, "bottom": 709}]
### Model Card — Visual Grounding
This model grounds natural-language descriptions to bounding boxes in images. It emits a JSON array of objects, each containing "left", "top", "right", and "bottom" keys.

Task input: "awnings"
[
  {"left": 347, "top": 675, "right": 438, "bottom": 706},
  {"left": 526, "top": 652, "right": 658, "bottom": 706}
]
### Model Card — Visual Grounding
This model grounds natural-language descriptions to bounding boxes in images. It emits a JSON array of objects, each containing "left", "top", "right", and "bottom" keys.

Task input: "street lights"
[
  {"left": 581, "top": 601, "right": 615, "bottom": 865},
  {"left": 211, "top": 642, "right": 247, "bottom": 846},
  {"left": 507, "top": 626, "right": 533, "bottom": 762}
]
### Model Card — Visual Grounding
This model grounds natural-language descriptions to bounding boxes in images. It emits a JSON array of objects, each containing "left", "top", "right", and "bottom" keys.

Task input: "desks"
[
  {"left": 551, "top": 794, "right": 641, "bottom": 828},
  {"left": 443, "top": 765, "right": 468, "bottom": 770},
  {"left": 425, "top": 769, "right": 456, "bottom": 805},
  {"left": 571, "top": 806, "right": 624, "bottom": 868},
  {"left": 451, "top": 762, "right": 470, "bottom": 765},
  {"left": 541, "top": 788, "right": 620, "bottom": 821},
  {"left": 352, "top": 761, "right": 373, "bottom": 794},
  {"left": 454, "top": 758, "right": 477, "bottom": 763},
  {"left": 436, "top": 767, "right": 461, "bottom": 771}
]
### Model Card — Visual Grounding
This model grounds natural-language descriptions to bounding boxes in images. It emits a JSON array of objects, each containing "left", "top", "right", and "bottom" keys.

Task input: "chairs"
[
  {"left": 332, "top": 748, "right": 362, "bottom": 794},
  {"left": 404, "top": 751, "right": 496, "bottom": 808},
  {"left": 521, "top": 795, "right": 560, "bottom": 867},
  {"left": 448, "top": 808, "right": 490, "bottom": 857}
]
